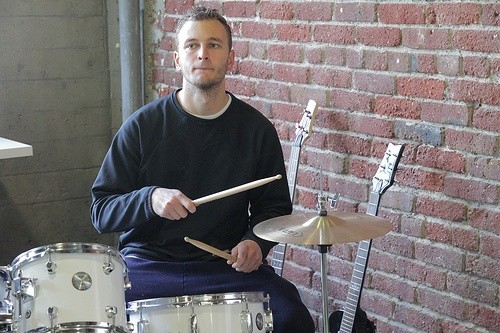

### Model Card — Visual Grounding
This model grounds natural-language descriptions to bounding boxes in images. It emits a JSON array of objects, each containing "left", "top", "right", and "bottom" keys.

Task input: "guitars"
[
  {"left": 268, "top": 100, "right": 318, "bottom": 277},
  {"left": 329, "top": 142, "right": 406, "bottom": 333}
]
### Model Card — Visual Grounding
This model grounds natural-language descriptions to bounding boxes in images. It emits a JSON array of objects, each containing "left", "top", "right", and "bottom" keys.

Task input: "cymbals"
[{"left": 252, "top": 211, "right": 395, "bottom": 246}]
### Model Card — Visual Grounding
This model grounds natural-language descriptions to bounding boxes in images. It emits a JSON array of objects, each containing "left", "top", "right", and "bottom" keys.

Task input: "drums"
[
  {"left": 127, "top": 290, "right": 274, "bottom": 333},
  {"left": 0, "top": 242, "right": 132, "bottom": 333}
]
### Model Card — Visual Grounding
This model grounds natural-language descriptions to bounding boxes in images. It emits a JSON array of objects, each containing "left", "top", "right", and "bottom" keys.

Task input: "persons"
[{"left": 89, "top": 5, "right": 316, "bottom": 332}]
